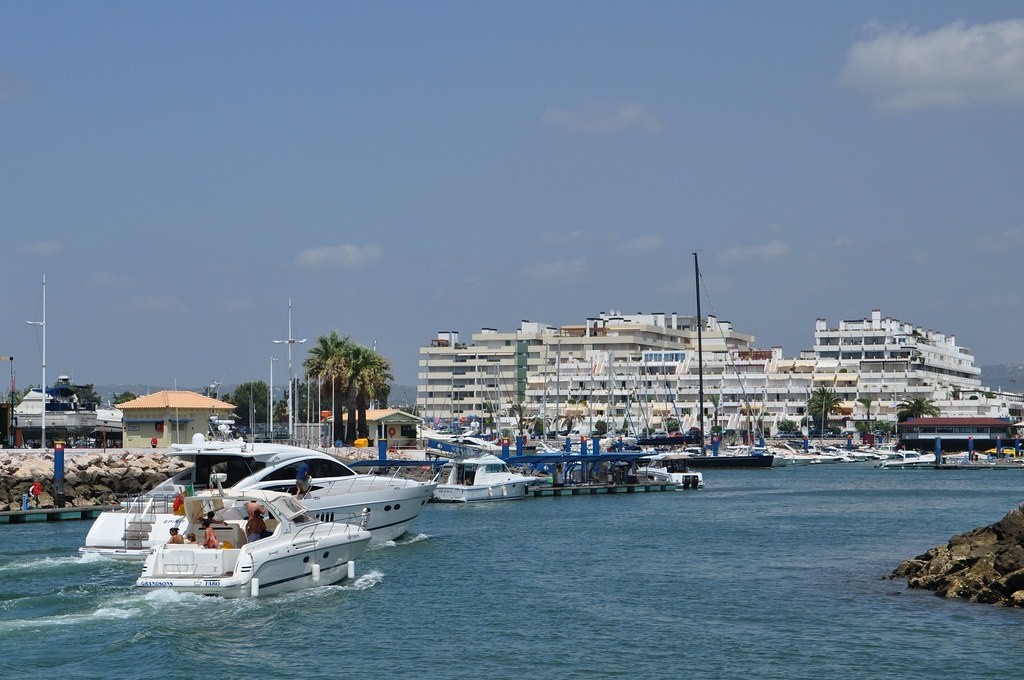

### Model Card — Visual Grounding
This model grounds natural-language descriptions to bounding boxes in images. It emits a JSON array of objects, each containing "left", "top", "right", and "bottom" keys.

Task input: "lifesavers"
[{"left": 389, "top": 427, "right": 396, "bottom": 436}]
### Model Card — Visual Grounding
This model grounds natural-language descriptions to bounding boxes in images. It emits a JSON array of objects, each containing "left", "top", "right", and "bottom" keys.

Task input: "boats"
[
  {"left": 429, "top": 453, "right": 537, "bottom": 505},
  {"left": 75, "top": 432, "right": 439, "bottom": 564},
  {"left": 629, "top": 466, "right": 706, "bottom": 492},
  {"left": 134, "top": 489, "right": 374, "bottom": 600},
  {"left": 415, "top": 252, "right": 1024, "bottom": 471}
]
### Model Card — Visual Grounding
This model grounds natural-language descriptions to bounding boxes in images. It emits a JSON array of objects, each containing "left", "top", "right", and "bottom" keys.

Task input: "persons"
[
  {"left": 199, "top": 511, "right": 228, "bottom": 549},
  {"left": 167, "top": 528, "right": 184, "bottom": 544},
  {"left": 245, "top": 500, "right": 269, "bottom": 543},
  {"left": 296, "top": 459, "right": 312, "bottom": 500},
  {"left": 186, "top": 534, "right": 199, "bottom": 545}
]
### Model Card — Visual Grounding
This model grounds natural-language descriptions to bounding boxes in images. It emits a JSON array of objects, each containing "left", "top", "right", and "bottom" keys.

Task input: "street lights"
[
  {"left": 272, "top": 297, "right": 307, "bottom": 436},
  {"left": 25, "top": 320, "right": 46, "bottom": 449},
  {"left": 269, "top": 356, "right": 279, "bottom": 432}
]
